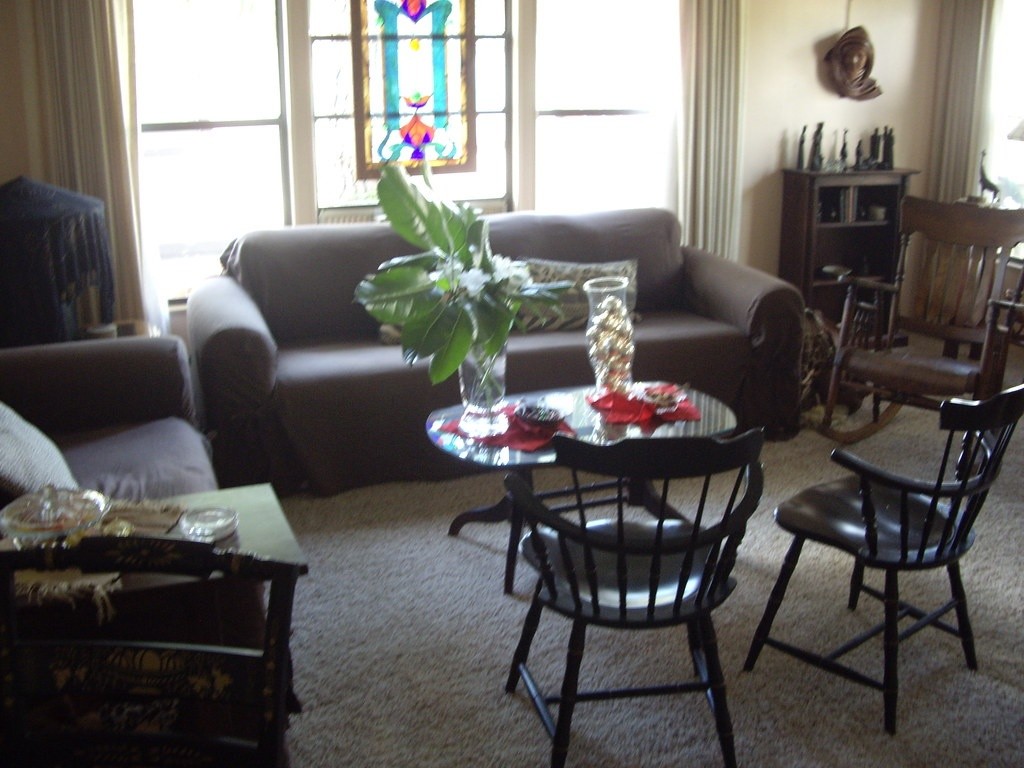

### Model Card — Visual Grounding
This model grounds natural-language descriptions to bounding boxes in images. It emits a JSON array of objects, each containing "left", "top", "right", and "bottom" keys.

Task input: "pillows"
[
  {"left": 512, "top": 252, "right": 643, "bottom": 332},
  {"left": 0, "top": 396, "right": 87, "bottom": 496}
]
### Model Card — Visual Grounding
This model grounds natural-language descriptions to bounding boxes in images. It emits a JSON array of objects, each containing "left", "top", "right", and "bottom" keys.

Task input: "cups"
[
  {"left": 181, "top": 505, "right": 240, "bottom": 551},
  {"left": 582, "top": 275, "right": 635, "bottom": 396}
]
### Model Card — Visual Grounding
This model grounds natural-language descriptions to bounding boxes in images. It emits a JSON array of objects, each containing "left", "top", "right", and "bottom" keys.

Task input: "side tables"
[
  {"left": 775, "top": 165, "right": 924, "bottom": 352},
  {"left": 84, "top": 471, "right": 304, "bottom": 723}
]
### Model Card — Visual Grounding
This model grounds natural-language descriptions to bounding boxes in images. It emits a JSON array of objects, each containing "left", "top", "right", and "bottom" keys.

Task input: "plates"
[{"left": 822, "top": 265, "right": 853, "bottom": 276}]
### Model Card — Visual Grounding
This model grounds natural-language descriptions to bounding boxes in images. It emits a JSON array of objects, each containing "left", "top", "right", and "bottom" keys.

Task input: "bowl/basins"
[{"left": 0, "top": 486, "right": 113, "bottom": 552}]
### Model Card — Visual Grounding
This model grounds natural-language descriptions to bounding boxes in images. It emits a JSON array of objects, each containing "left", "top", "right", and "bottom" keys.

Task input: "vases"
[
  {"left": 580, "top": 271, "right": 641, "bottom": 403},
  {"left": 456, "top": 341, "right": 509, "bottom": 439}
]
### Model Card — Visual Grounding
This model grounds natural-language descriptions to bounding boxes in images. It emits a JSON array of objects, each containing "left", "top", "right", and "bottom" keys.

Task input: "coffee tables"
[{"left": 423, "top": 381, "right": 740, "bottom": 595}]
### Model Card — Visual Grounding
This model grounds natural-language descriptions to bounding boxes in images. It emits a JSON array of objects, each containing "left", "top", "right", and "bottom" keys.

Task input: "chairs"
[
  {"left": 0, "top": 543, "right": 314, "bottom": 768},
  {"left": 739, "top": 382, "right": 1024, "bottom": 737},
  {"left": 817, "top": 193, "right": 1024, "bottom": 453},
  {"left": 500, "top": 418, "right": 768, "bottom": 768}
]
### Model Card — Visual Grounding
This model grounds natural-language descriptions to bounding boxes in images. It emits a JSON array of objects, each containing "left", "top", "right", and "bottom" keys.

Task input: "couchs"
[
  {"left": 190, "top": 198, "right": 809, "bottom": 512},
  {"left": 0, "top": 331, "right": 268, "bottom": 749}
]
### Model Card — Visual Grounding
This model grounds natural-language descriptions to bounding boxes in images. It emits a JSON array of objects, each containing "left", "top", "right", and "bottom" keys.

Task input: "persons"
[{"left": 823, "top": 26, "right": 882, "bottom": 101}]
